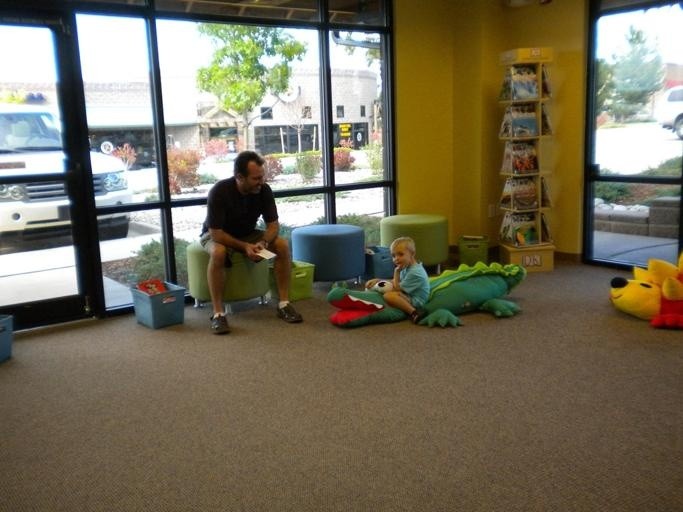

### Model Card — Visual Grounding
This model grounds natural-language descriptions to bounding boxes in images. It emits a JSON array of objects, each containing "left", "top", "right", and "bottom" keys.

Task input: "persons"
[
  {"left": 200, "top": 151, "right": 302, "bottom": 335},
  {"left": 369, "top": 237, "right": 430, "bottom": 323}
]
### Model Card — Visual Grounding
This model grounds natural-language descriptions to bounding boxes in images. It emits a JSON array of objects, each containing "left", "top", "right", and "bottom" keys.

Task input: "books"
[{"left": 496, "top": 65, "right": 555, "bottom": 247}]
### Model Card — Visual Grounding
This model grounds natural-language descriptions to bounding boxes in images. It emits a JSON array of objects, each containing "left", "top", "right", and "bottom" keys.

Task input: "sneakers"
[
  {"left": 209, "top": 314, "right": 232, "bottom": 334},
  {"left": 275, "top": 302, "right": 302, "bottom": 323},
  {"left": 411, "top": 309, "right": 420, "bottom": 324}
]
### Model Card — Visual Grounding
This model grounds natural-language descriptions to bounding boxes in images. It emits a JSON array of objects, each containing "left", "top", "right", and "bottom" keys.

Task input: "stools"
[
  {"left": 291, "top": 224, "right": 366, "bottom": 283},
  {"left": 185, "top": 241, "right": 269, "bottom": 314},
  {"left": 380, "top": 213, "right": 450, "bottom": 276}
]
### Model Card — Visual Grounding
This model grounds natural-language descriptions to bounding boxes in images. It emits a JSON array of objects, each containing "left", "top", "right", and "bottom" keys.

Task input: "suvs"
[
  {"left": 0, "top": 103, "right": 130, "bottom": 239},
  {"left": 653, "top": 85, "right": 682, "bottom": 138}
]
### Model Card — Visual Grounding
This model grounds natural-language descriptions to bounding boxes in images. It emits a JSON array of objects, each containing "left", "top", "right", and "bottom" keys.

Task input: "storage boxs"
[
  {"left": 270, "top": 261, "right": 315, "bottom": 299},
  {"left": 455, "top": 234, "right": 492, "bottom": 268},
  {"left": 0, "top": 314, "right": 15, "bottom": 360},
  {"left": 129, "top": 277, "right": 185, "bottom": 327},
  {"left": 364, "top": 245, "right": 393, "bottom": 280}
]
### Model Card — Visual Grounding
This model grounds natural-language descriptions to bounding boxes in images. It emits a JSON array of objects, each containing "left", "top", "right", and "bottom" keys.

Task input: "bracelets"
[{"left": 260, "top": 237, "right": 269, "bottom": 249}]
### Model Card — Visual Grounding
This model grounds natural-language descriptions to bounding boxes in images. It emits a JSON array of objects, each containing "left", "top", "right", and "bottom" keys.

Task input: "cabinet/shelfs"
[{"left": 497, "top": 58, "right": 555, "bottom": 274}]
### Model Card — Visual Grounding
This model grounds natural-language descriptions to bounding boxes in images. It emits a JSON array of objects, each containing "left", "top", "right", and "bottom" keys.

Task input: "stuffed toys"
[
  {"left": 323, "top": 262, "right": 526, "bottom": 327},
  {"left": 607, "top": 255, "right": 682, "bottom": 328}
]
